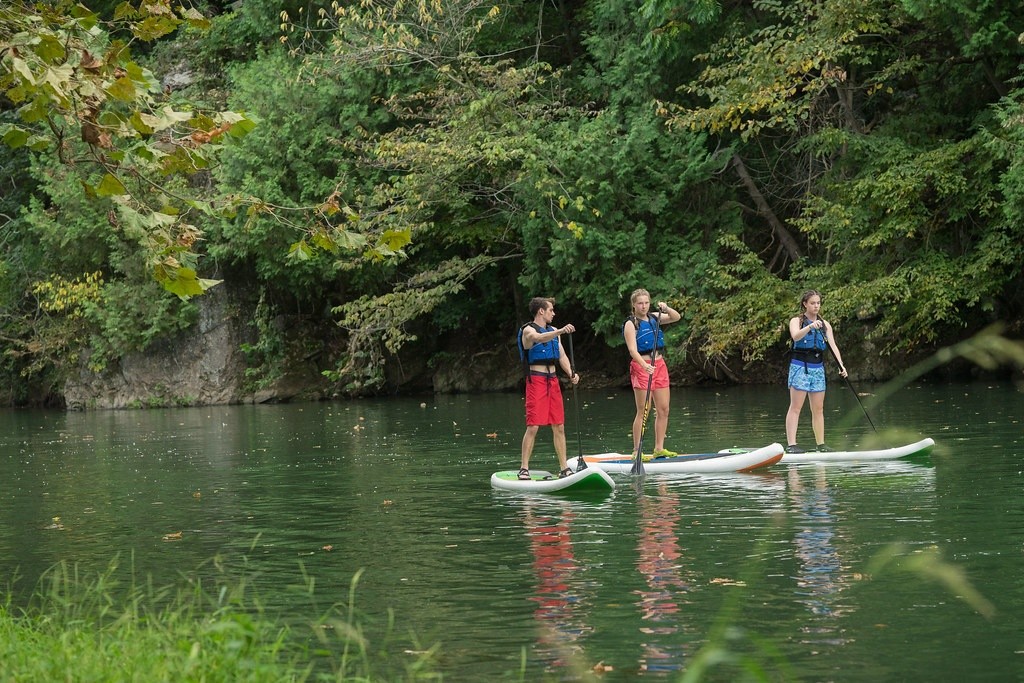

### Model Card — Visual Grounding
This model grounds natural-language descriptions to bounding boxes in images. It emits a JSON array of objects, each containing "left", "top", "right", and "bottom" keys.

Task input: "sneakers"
[
  {"left": 786, "top": 444, "right": 806, "bottom": 454},
  {"left": 816, "top": 444, "right": 836, "bottom": 453}
]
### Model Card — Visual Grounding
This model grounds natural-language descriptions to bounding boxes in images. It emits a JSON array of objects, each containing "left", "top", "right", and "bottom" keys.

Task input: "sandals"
[
  {"left": 558, "top": 468, "right": 575, "bottom": 479},
  {"left": 517, "top": 468, "right": 531, "bottom": 480},
  {"left": 631, "top": 452, "right": 651, "bottom": 462},
  {"left": 653, "top": 448, "right": 677, "bottom": 458}
]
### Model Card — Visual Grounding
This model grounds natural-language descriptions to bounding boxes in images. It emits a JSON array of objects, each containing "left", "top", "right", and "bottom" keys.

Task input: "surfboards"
[
  {"left": 717, "top": 434, "right": 935, "bottom": 465},
  {"left": 489, "top": 467, "right": 615, "bottom": 495},
  {"left": 567, "top": 442, "right": 786, "bottom": 475}
]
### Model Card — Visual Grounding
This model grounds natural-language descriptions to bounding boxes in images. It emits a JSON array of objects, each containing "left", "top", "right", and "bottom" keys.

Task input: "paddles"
[
  {"left": 628, "top": 306, "right": 664, "bottom": 479},
  {"left": 566, "top": 325, "right": 590, "bottom": 472},
  {"left": 818, "top": 320, "right": 882, "bottom": 434}
]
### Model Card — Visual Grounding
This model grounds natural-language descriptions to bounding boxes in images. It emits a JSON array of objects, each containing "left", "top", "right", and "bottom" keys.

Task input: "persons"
[
  {"left": 518, "top": 296, "right": 581, "bottom": 481},
  {"left": 785, "top": 289, "right": 849, "bottom": 454},
  {"left": 623, "top": 288, "right": 681, "bottom": 462}
]
